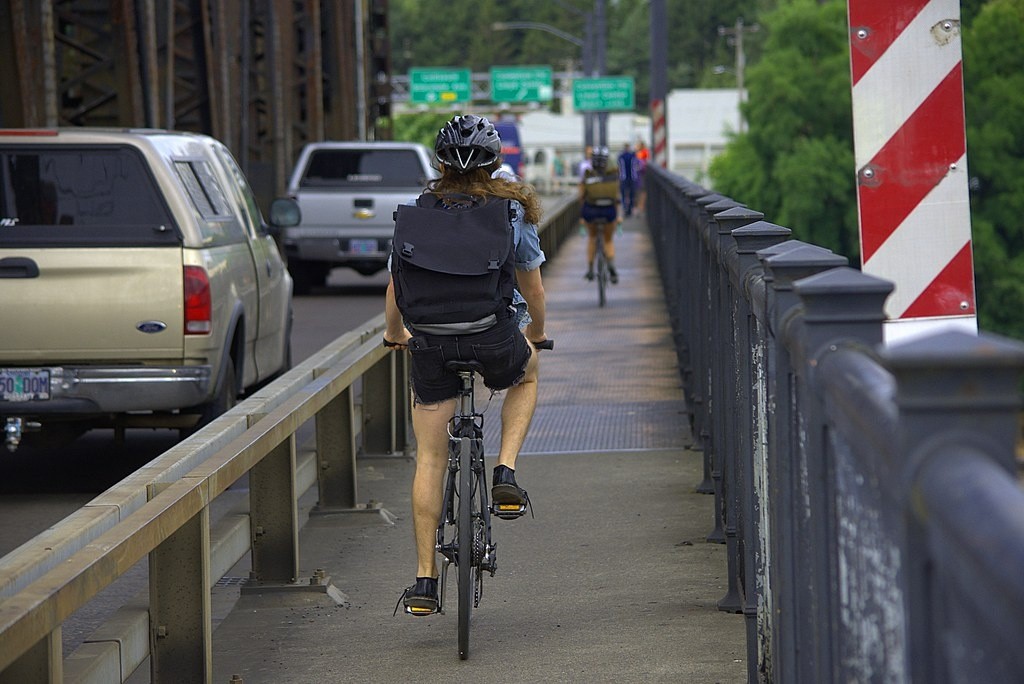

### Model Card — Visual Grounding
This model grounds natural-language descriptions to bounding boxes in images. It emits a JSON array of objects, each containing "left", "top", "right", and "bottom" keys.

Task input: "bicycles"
[
  {"left": 584, "top": 218, "right": 619, "bottom": 308},
  {"left": 382, "top": 336, "right": 555, "bottom": 659}
]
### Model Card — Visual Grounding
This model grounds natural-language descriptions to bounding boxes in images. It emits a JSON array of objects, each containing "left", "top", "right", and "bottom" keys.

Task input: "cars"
[
  {"left": 0, "top": 127, "right": 295, "bottom": 453},
  {"left": 278, "top": 142, "right": 444, "bottom": 299}
]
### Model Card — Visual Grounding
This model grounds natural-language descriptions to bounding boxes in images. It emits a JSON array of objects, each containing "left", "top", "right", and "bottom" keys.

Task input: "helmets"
[
  {"left": 592, "top": 146, "right": 609, "bottom": 157},
  {"left": 434, "top": 115, "right": 502, "bottom": 174}
]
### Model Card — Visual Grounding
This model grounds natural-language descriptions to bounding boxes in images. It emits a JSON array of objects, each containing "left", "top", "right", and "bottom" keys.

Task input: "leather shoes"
[
  {"left": 393, "top": 576, "right": 441, "bottom": 617},
  {"left": 491, "top": 465, "right": 535, "bottom": 520}
]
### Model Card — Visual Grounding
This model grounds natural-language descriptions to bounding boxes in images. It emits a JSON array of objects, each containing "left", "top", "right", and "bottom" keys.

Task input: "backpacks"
[
  {"left": 390, "top": 193, "right": 516, "bottom": 325},
  {"left": 584, "top": 167, "right": 621, "bottom": 206}
]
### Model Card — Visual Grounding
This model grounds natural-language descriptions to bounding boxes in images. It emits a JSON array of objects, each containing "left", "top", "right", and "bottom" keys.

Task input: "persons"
[
  {"left": 580, "top": 146, "right": 624, "bottom": 283},
  {"left": 618, "top": 141, "right": 649, "bottom": 218},
  {"left": 578, "top": 145, "right": 593, "bottom": 236},
  {"left": 383, "top": 115, "right": 546, "bottom": 610}
]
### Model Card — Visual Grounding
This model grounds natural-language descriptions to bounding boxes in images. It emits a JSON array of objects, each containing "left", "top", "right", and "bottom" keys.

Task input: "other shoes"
[
  {"left": 585, "top": 273, "right": 594, "bottom": 281},
  {"left": 624, "top": 207, "right": 632, "bottom": 217},
  {"left": 607, "top": 264, "right": 618, "bottom": 284}
]
